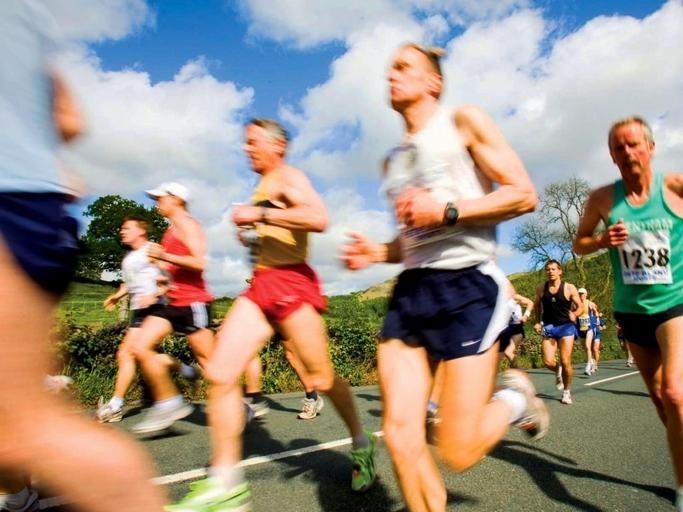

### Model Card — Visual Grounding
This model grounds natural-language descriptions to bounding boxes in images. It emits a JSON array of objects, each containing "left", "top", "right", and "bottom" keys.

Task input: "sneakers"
[
  {"left": 555, "top": 368, "right": 564, "bottom": 391},
  {"left": 0, "top": 485, "right": 39, "bottom": 512},
  {"left": 584, "top": 359, "right": 598, "bottom": 376},
  {"left": 497, "top": 368, "right": 551, "bottom": 445},
  {"left": 297, "top": 393, "right": 324, "bottom": 420},
  {"left": 244, "top": 398, "right": 270, "bottom": 424},
  {"left": 129, "top": 393, "right": 195, "bottom": 434},
  {"left": 187, "top": 366, "right": 205, "bottom": 399},
  {"left": 161, "top": 472, "right": 255, "bottom": 512},
  {"left": 94, "top": 402, "right": 123, "bottom": 423},
  {"left": 561, "top": 388, "right": 572, "bottom": 404},
  {"left": 625, "top": 358, "right": 635, "bottom": 367},
  {"left": 349, "top": 429, "right": 379, "bottom": 492}
]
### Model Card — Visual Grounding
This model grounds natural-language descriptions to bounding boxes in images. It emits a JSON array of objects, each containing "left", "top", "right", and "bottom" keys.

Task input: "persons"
[
  {"left": 336, "top": 41, "right": 548, "bottom": 512},
  {"left": 238, "top": 338, "right": 325, "bottom": 425},
  {"left": 0, "top": 23, "right": 173, "bottom": 510},
  {"left": 572, "top": 113, "right": 681, "bottom": 512},
  {"left": 123, "top": 182, "right": 252, "bottom": 441},
  {"left": 94, "top": 214, "right": 202, "bottom": 424},
  {"left": 497, "top": 255, "right": 634, "bottom": 410},
  {"left": 165, "top": 119, "right": 380, "bottom": 510},
  {"left": 425, "top": 359, "right": 446, "bottom": 447}
]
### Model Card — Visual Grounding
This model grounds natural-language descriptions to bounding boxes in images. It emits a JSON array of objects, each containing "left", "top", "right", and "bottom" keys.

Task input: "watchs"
[{"left": 443, "top": 200, "right": 459, "bottom": 227}]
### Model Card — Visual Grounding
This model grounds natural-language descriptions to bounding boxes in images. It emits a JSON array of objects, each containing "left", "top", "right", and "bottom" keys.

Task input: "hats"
[
  {"left": 144, "top": 181, "right": 192, "bottom": 204},
  {"left": 578, "top": 288, "right": 587, "bottom": 295}
]
